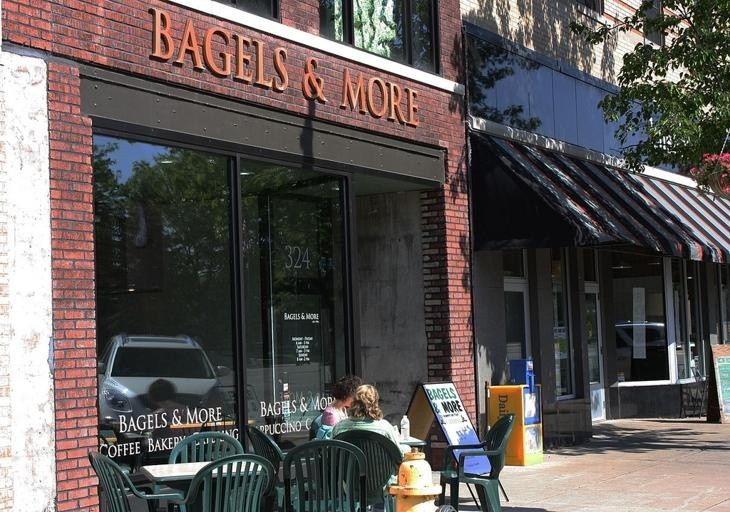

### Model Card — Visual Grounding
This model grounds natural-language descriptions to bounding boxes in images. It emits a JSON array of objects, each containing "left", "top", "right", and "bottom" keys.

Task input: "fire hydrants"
[{"left": 389, "top": 447, "right": 443, "bottom": 512}]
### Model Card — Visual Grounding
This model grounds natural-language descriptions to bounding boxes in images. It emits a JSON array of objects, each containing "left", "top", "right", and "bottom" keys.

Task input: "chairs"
[
  {"left": 437, "top": 413, "right": 517, "bottom": 512},
  {"left": 86, "top": 423, "right": 402, "bottom": 512}
]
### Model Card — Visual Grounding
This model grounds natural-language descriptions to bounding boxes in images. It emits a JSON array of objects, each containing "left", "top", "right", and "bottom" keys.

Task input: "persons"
[{"left": 310, "top": 376, "right": 412, "bottom": 497}]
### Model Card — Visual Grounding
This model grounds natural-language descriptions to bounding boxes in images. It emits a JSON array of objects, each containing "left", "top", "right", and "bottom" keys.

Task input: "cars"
[
  {"left": 98, "top": 331, "right": 228, "bottom": 428},
  {"left": 613, "top": 318, "right": 668, "bottom": 354}
]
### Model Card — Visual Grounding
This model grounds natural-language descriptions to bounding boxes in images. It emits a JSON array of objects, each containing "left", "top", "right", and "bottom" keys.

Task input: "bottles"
[{"left": 401, "top": 415, "right": 411, "bottom": 439}]
